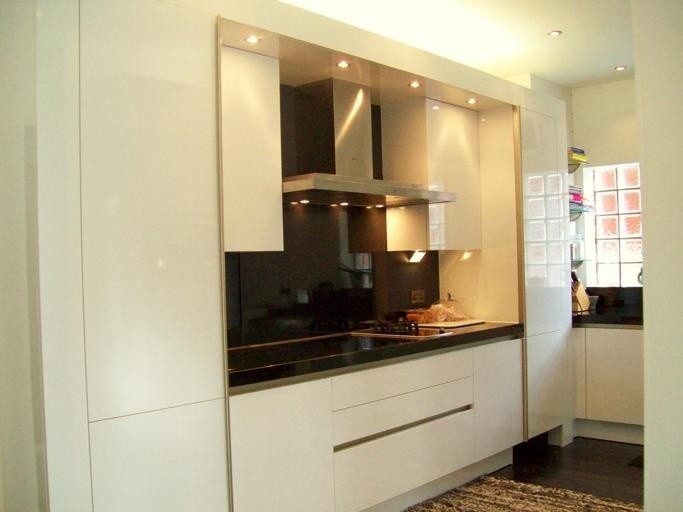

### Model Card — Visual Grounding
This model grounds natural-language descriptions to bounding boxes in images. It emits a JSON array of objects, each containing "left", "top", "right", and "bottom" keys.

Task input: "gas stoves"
[{"left": 351, "top": 310, "right": 454, "bottom": 343}]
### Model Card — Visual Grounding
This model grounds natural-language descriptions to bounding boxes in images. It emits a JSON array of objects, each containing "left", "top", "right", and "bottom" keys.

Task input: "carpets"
[{"left": 402, "top": 475, "right": 643, "bottom": 512}]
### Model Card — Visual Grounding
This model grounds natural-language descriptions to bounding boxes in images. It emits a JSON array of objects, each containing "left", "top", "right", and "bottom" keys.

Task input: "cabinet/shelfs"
[
  {"left": 379, "top": 96, "right": 481, "bottom": 253},
  {"left": 571, "top": 328, "right": 643, "bottom": 446},
  {"left": 0, "top": 0, "right": 231, "bottom": 511},
  {"left": 438, "top": 107, "right": 573, "bottom": 449},
  {"left": 220, "top": 44, "right": 285, "bottom": 253}
]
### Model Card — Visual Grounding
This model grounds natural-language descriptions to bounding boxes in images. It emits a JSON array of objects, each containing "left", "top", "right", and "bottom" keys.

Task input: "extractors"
[{"left": 283, "top": 76, "right": 457, "bottom": 209}]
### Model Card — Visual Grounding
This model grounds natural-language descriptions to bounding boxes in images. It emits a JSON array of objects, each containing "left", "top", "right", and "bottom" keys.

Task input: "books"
[{"left": 568, "top": 145, "right": 594, "bottom": 214}]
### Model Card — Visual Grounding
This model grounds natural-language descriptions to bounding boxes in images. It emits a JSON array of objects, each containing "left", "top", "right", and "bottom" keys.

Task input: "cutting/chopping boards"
[{"left": 417, "top": 318, "right": 485, "bottom": 329}]
